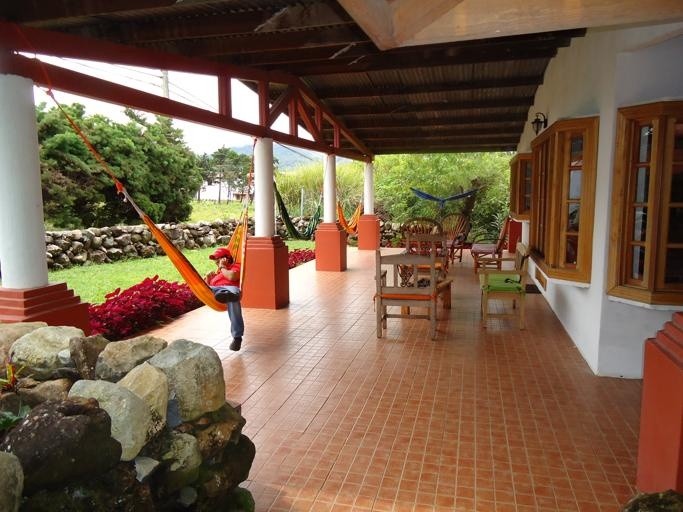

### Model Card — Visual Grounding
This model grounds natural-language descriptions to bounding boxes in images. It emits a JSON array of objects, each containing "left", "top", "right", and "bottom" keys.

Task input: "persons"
[{"left": 202, "top": 248, "right": 244, "bottom": 351}]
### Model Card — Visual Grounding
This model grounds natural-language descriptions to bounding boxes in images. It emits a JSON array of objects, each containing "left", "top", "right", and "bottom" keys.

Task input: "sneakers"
[
  {"left": 215, "top": 292, "right": 239, "bottom": 303},
  {"left": 230, "top": 337, "right": 242, "bottom": 351}
]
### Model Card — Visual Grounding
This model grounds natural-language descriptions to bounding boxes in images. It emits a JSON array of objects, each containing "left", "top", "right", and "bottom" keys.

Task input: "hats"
[{"left": 209, "top": 248, "right": 232, "bottom": 259}]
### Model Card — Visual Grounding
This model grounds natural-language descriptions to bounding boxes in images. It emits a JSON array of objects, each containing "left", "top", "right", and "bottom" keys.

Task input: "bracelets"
[{"left": 220, "top": 266, "right": 226, "bottom": 270}]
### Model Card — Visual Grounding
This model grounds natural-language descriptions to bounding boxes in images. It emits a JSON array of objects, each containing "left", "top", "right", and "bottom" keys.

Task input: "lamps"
[{"left": 530, "top": 112, "right": 548, "bottom": 134}]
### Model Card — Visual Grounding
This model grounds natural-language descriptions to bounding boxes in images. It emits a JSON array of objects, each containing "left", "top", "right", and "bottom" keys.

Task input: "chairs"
[{"left": 373, "top": 212, "right": 528, "bottom": 340}]
